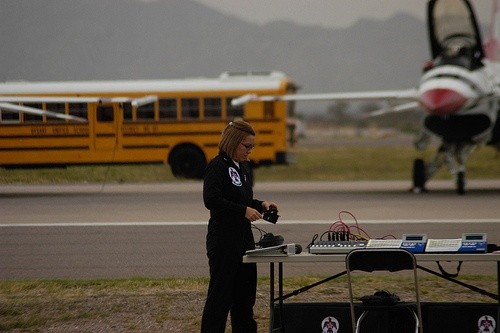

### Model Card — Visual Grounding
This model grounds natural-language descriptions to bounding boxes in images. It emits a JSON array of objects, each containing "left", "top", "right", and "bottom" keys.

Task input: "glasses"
[{"left": 241, "top": 143, "right": 255, "bottom": 151}]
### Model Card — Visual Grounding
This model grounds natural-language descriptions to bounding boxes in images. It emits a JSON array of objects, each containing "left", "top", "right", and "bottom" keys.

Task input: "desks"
[{"left": 242, "top": 242, "right": 500, "bottom": 333}]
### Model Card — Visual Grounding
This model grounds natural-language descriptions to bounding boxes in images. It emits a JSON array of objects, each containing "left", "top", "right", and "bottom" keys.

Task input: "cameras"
[{"left": 263, "top": 205, "right": 279, "bottom": 224}]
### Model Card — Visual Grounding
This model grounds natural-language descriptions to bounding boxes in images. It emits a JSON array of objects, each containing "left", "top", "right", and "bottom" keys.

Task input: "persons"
[{"left": 199, "top": 119, "right": 278, "bottom": 333}]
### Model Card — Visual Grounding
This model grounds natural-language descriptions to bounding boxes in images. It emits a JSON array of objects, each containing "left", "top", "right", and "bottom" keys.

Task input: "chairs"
[{"left": 345, "top": 246, "right": 424, "bottom": 333}]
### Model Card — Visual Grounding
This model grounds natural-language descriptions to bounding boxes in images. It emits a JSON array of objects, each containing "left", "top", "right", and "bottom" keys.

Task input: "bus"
[{"left": 0, "top": 70, "right": 298, "bottom": 181}]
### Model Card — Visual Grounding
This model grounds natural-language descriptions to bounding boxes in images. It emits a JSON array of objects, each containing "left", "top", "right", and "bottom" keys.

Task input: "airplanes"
[{"left": 230, "top": 1, "right": 500, "bottom": 194}]
